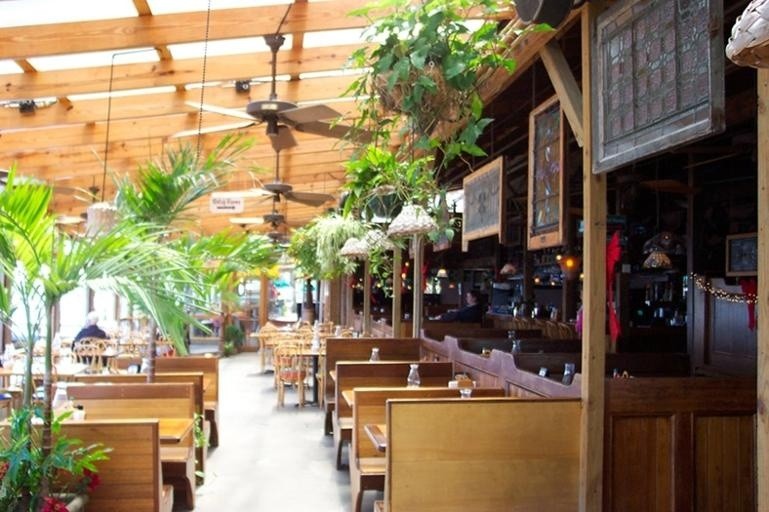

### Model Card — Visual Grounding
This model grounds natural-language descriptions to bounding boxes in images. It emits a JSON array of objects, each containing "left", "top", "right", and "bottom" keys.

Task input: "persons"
[
  {"left": 70, "top": 312, "right": 110, "bottom": 365},
  {"left": 433, "top": 287, "right": 485, "bottom": 323},
  {"left": 574, "top": 290, "right": 583, "bottom": 340}
]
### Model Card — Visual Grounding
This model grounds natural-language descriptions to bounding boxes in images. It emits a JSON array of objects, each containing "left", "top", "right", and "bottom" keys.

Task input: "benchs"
[{"left": 1, "top": 358, "right": 220, "bottom": 511}]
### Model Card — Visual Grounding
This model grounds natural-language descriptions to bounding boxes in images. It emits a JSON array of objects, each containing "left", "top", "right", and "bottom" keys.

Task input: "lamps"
[
  {"left": 387, "top": 117, "right": 438, "bottom": 238},
  {"left": 264, "top": 114, "right": 278, "bottom": 137},
  {"left": 337, "top": 203, "right": 368, "bottom": 257},
  {"left": 85, "top": 56, "right": 117, "bottom": 237}
]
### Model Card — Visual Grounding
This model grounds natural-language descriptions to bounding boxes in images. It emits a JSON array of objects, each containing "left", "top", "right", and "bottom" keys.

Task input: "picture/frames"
[{"left": 526, "top": 93, "right": 569, "bottom": 251}]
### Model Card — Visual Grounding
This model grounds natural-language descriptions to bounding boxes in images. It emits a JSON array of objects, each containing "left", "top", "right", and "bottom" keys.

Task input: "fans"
[
  {"left": 211, "top": 152, "right": 331, "bottom": 244},
  {"left": 171, "top": 3, "right": 374, "bottom": 153}
]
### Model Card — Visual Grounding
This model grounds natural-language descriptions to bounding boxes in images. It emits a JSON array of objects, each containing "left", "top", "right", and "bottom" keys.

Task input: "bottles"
[
  {"left": 508, "top": 330, "right": 516, "bottom": 342},
  {"left": 510, "top": 339, "right": 523, "bottom": 353},
  {"left": 459, "top": 388, "right": 472, "bottom": 398},
  {"left": 310, "top": 332, "right": 321, "bottom": 353},
  {"left": 656, "top": 298, "right": 666, "bottom": 326},
  {"left": 407, "top": 364, "right": 422, "bottom": 387},
  {"left": 562, "top": 362, "right": 575, "bottom": 385},
  {"left": 140, "top": 357, "right": 150, "bottom": 373},
  {"left": 312, "top": 320, "right": 321, "bottom": 331},
  {"left": 52, "top": 381, "right": 71, "bottom": 421},
  {"left": 369, "top": 347, "right": 380, "bottom": 361},
  {"left": 334, "top": 325, "right": 342, "bottom": 337},
  {"left": 513, "top": 299, "right": 562, "bottom": 323}
]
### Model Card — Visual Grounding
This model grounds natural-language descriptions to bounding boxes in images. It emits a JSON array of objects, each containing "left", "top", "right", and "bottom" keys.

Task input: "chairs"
[
  {"left": 247, "top": 318, "right": 378, "bottom": 411},
  {"left": 0, "top": 314, "right": 176, "bottom": 419}
]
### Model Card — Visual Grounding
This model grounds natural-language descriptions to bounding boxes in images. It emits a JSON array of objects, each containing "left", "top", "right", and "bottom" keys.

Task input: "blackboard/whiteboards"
[
  {"left": 461, "top": 95, "right": 569, "bottom": 251},
  {"left": 591, "top": 0, "right": 725, "bottom": 175}
]
